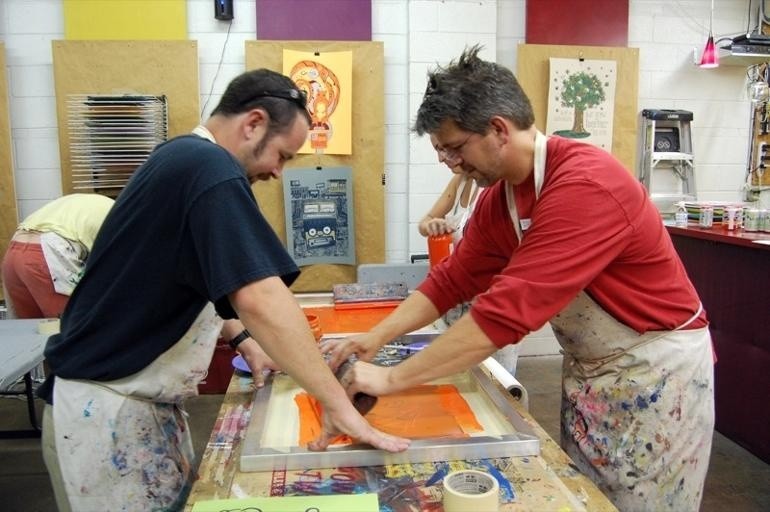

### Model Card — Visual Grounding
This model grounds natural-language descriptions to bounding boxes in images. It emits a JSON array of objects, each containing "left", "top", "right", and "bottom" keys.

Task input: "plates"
[
  {"left": 230, "top": 354, "right": 248, "bottom": 373},
  {"left": 673, "top": 200, "right": 746, "bottom": 223}
]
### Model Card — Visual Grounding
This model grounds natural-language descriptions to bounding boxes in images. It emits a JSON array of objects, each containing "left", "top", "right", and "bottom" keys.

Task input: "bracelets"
[{"left": 230, "top": 327, "right": 253, "bottom": 350}]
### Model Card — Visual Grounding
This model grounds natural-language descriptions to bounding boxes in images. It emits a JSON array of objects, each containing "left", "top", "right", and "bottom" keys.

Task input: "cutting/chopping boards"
[{"left": 237, "top": 332, "right": 541, "bottom": 472}]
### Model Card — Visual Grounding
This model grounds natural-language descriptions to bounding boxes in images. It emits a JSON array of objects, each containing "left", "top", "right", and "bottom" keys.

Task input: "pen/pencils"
[{"left": 425, "top": 464, "right": 449, "bottom": 487}]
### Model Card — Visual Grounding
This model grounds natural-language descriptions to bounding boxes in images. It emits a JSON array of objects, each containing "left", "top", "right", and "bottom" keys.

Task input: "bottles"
[
  {"left": 428, "top": 233, "right": 453, "bottom": 271},
  {"left": 746, "top": 81, "right": 769, "bottom": 110}
]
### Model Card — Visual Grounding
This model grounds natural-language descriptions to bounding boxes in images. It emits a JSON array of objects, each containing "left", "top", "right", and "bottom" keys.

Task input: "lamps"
[{"left": 698, "top": 0, "right": 721, "bottom": 68}]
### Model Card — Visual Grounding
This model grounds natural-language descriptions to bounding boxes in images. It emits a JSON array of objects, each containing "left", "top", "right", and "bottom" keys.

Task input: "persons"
[
  {"left": 422, "top": 135, "right": 522, "bottom": 377},
  {"left": 41, "top": 67, "right": 410, "bottom": 512},
  {"left": 0, "top": 192, "right": 117, "bottom": 318},
  {"left": 315, "top": 44, "right": 718, "bottom": 512}
]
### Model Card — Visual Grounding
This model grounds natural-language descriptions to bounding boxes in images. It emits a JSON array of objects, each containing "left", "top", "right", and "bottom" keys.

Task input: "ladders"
[{"left": 642, "top": 108, "right": 697, "bottom": 202}]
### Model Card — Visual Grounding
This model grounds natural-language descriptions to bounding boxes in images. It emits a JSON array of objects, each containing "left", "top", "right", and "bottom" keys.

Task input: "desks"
[
  {"left": 180, "top": 302, "right": 621, "bottom": 512},
  {"left": 0, "top": 318, "right": 61, "bottom": 439}
]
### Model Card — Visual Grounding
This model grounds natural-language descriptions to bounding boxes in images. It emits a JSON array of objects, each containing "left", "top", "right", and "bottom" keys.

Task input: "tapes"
[{"left": 443, "top": 469, "right": 499, "bottom": 512}]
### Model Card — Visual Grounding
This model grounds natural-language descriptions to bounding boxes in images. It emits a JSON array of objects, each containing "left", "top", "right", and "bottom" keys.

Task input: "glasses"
[
  {"left": 236, "top": 86, "right": 308, "bottom": 109},
  {"left": 440, "top": 132, "right": 476, "bottom": 161}
]
